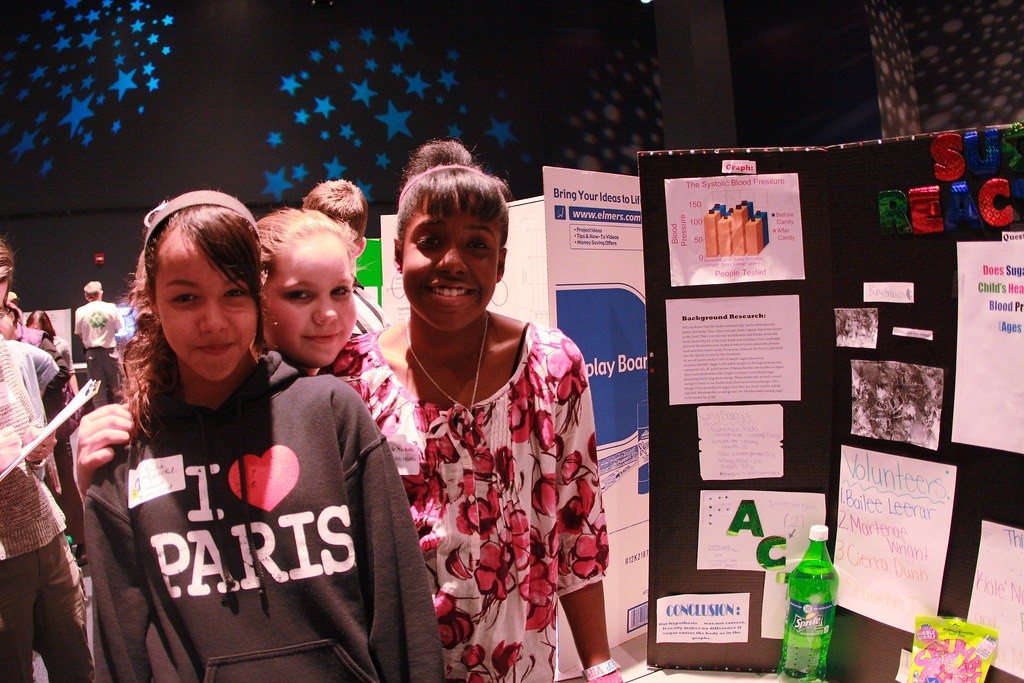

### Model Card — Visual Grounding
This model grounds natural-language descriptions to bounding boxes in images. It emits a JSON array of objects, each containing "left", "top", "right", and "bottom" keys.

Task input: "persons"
[
  {"left": 316, "top": 142, "right": 627, "bottom": 683},
  {"left": 0, "top": 178, "right": 446, "bottom": 683}
]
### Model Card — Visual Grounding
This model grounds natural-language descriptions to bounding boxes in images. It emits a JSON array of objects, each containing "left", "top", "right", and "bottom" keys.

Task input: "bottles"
[{"left": 777, "top": 525, "right": 838, "bottom": 683}]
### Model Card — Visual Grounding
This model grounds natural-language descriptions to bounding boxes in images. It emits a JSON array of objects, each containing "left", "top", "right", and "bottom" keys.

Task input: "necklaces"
[{"left": 407, "top": 314, "right": 488, "bottom": 405}]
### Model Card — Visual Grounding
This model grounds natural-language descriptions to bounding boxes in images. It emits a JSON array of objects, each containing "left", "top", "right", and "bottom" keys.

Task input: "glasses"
[{"left": 0, "top": 311, "right": 8, "bottom": 320}]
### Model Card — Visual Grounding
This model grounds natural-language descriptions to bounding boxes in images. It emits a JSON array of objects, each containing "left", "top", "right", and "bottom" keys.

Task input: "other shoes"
[{"left": 74, "top": 545, "right": 89, "bottom": 566}]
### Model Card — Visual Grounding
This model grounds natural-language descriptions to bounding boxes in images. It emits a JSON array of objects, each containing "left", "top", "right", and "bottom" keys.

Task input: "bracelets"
[
  {"left": 588, "top": 671, "right": 624, "bottom": 683},
  {"left": 582, "top": 658, "right": 621, "bottom": 680}
]
